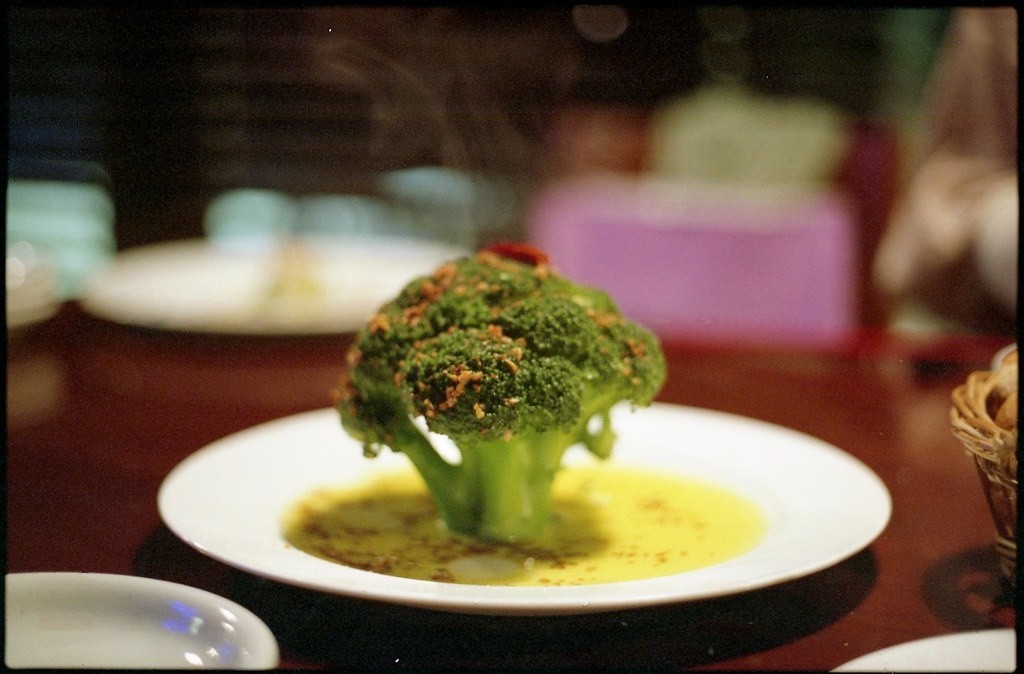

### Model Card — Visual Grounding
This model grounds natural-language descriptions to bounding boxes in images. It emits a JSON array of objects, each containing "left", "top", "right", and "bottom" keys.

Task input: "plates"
[
  {"left": 154, "top": 394, "right": 896, "bottom": 619},
  {"left": 4, "top": 570, "right": 280, "bottom": 671},
  {"left": 832, "top": 628, "right": 1019, "bottom": 673},
  {"left": 78, "top": 233, "right": 477, "bottom": 334}
]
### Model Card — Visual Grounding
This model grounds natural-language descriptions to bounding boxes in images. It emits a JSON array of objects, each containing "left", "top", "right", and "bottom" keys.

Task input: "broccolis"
[{"left": 330, "top": 252, "right": 667, "bottom": 544}]
